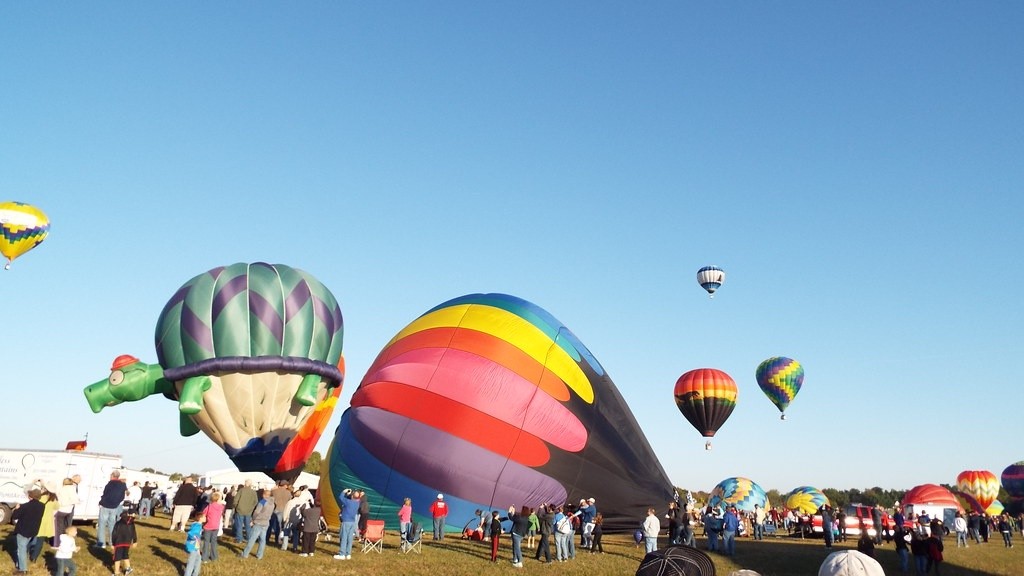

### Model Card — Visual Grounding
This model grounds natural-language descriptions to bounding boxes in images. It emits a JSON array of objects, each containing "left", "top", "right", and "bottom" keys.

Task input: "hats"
[
  {"left": 437, "top": 494, "right": 443, "bottom": 499},
  {"left": 817, "top": 549, "right": 885, "bottom": 576},
  {"left": 44, "top": 481, "right": 57, "bottom": 493},
  {"left": 278, "top": 480, "right": 289, "bottom": 485},
  {"left": 28, "top": 489, "right": 41, "bottom": 500},
  {"left": 635, "top": 544, "right": 715, "bottom": 576},
  {"left": 587, "top": 498, "right": 595, "bottom": 504}
]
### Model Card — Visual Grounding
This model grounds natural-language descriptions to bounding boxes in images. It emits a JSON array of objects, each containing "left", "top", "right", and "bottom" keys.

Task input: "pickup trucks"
[{"left": 801, "top": 504, "right": 925, "bottom": 542}]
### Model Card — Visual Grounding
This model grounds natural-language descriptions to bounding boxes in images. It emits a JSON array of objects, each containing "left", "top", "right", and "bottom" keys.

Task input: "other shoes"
[
  {"left": 600, "top": 551, "right": 605, "bottom": 555},
  {"left": 299, "top": 553, "right": 314, "bottom": 557},
  {"left": 963, "top": 545, "right": 970, "bottom": 548},
  {"left": 99, "top": 543, "right": 106, "bottom": 549},
  {"left": 513, "top": 563, "right": 523, "bottom": 568},
  {"left": 124, "top": 568, "right": 133, "bottom": 575},
  {"left": 17, "top": 571, "right": 27, "bottom": 574},
  {"left": 50, "top": 547, "right": 59, "bottom": 549},
  {"left": 579, "top": 545, "right": 592, "bottom": 549},
  {"left": 588, "top": 552, "right": 595, "bottom": 554},
  {"left": 510, "top": 560, "right": 518, "bottom": 563},
  {"left": 334, "top": 554, "right": 352, "bottom": 559},
  {"left": 1010, "top": 545, "right": 1013, "bottom": 548}
]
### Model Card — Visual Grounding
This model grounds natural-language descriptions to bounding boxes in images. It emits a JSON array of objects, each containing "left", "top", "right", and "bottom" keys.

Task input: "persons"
[
  {"left": 54, "top": 526, "right": 81, "bottom": 576},
  {"left": 635, "top": 544, "right": 717, "bottom": 576},
  {"left": 817, "top": 550, "right": 886, "bottom": 576},
  {"left": 463, "top": 486, "right": 766, "bottom": 567},
  {"left": 768, "top": 504, "right": 847, "bottom": 549},
  {"left": 50, "top": 474, "right": 81, "bottom": 548},
  {"left": 870, "top": 502, "right": 945, "bottom": 576},
  {"left": 728, "top": 569, "right": 762, "bottom": 576},
  {"left": 170, "top": 476, "right": 449, "bottom": 576},
  {"left": 954, "top": 510, "right": 1024, "bottom": 548},
  {"left": 13, "top": 478, "right": 60, "bottom": 574},
  {"left": 93, "top": 470, "right": 158, "bottom": 548},
  {"left": 112, "top": 511, "right": 137, "bottom": 576}
]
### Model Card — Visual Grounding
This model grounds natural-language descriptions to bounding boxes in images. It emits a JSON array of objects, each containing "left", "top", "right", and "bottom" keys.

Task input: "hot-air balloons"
[
  {"left": 898, "top": 483, "right": 967, "bottom": 529},
  {"left": 951, "top": 470, "right": 1005, "bottom": 516},
  {"left": 316, "top": 293, "right": 686, "bottom": 535},
  {"left": 756, "top": 356, "right": 804, "bottom": 420},
  {"left": 84, "top": 262, "right": 345, "bottom": 487},
  {"left": 786, "top": 486, "right": 830, "bottom": 514},
  {"left": 1001, "top": 461, "right": 1024, "bottom": 502},
  {"left": 0, "top": 201, "right": 51, "bottom": 271},
  {"left": 708, "top": 477, "right": 772, "bottom": 524},
  {"left": 674, "top": 368, "right": 739, "bottom": 450},
  {"left": 697, "top": 265, "right": 726, "bottom": 298}
]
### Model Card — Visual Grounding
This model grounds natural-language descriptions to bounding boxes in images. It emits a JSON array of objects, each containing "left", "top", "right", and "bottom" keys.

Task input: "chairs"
[
  {"left": 360, "top": 520, "right": 386, "bottom": 555},
  {"left": 402, "top": 522, "right": 425, "bottom": 554}
]
